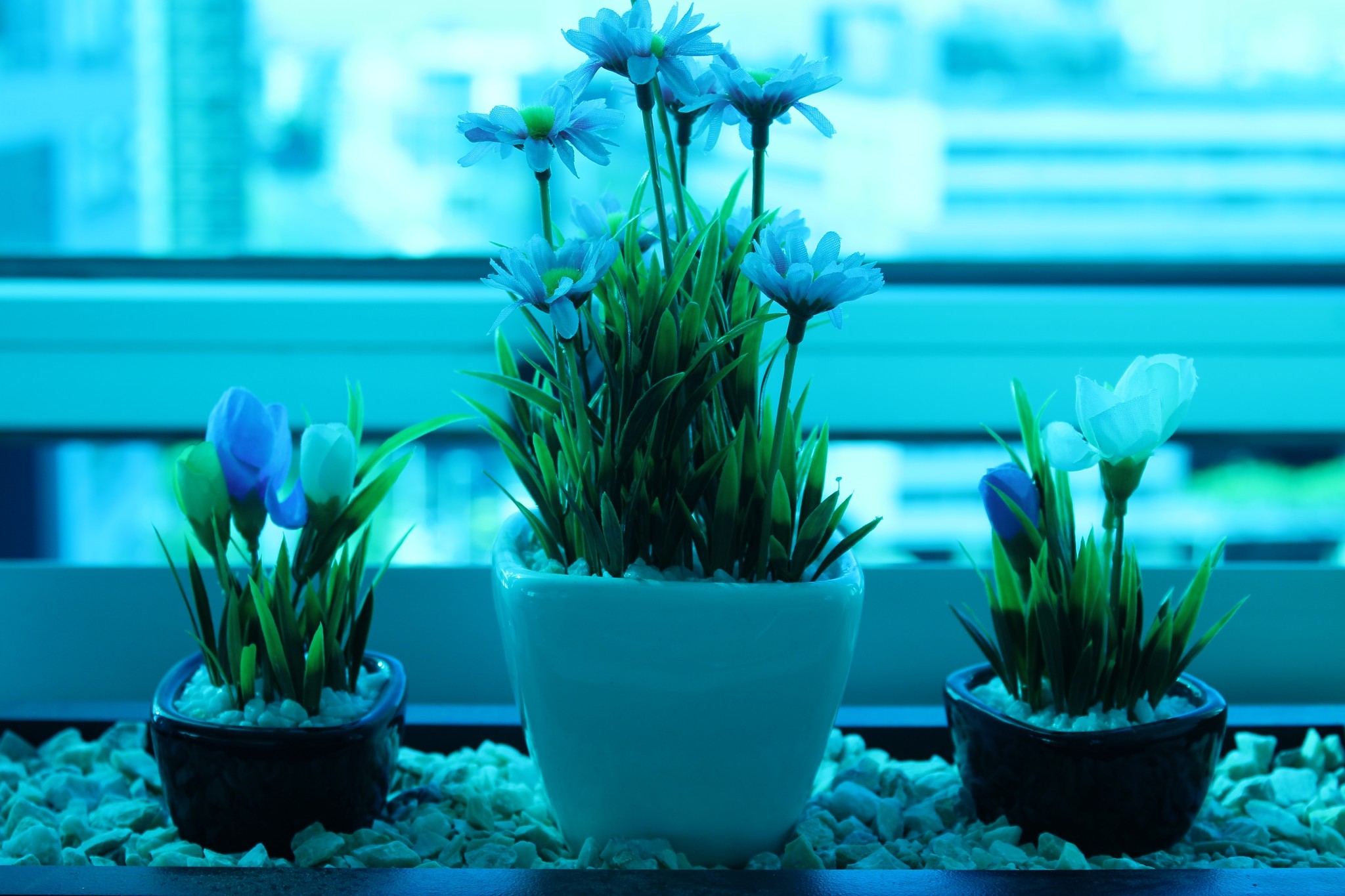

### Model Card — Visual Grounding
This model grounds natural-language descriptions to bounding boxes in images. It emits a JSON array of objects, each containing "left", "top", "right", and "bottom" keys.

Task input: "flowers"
[
  {"left": 150, "top": 381, "right": 477, "bottom": 711},
  {"left": 949, "top": 353, "right": 1252, "bottom": 717},
  {"left": 452, "top": 0, "right": 886, "bottom": 583}
]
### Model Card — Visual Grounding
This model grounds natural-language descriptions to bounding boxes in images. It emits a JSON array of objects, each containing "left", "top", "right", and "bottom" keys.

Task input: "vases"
[
  {"left": 491, "top": 508, "right": 864, "bottom": 868},
  {"left": 148, "top": 650, "right": 406, "bottom": 863},
  {"left": 942, "top": 660, "right": 1228, "bottom": 858}
]
